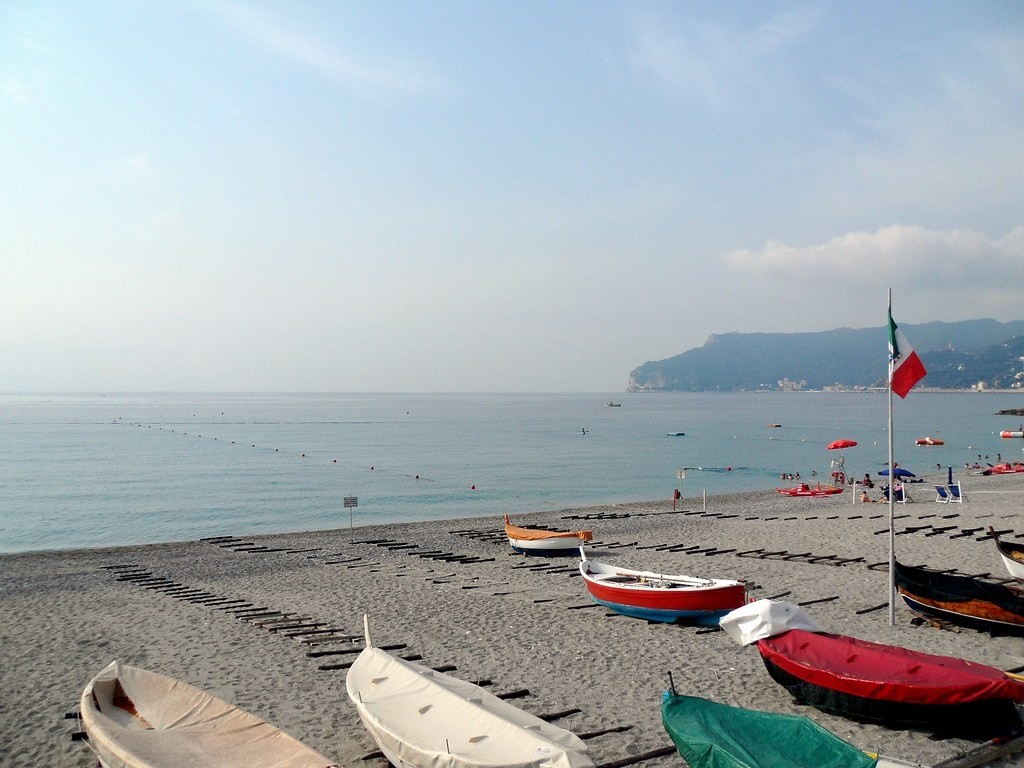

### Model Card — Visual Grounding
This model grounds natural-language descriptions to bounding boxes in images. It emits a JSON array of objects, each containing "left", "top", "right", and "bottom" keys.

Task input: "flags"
[{"left": 886, "top": 311, "right": 927, "bottom": 399}]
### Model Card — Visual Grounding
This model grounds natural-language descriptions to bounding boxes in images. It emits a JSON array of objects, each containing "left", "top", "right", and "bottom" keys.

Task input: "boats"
[
  {"left": 578, "top": 544, "right": 755, "bottom": 626},
  {"left": 749, "top": 596, "right": 1024, "bottom": 726},
  {"left": 660, "top": 670, "right": 879, "bottom": 768},
  {"left": 916, "top": 439, "right": 943, "bottom": 446},
  {"left": 604, "top": 402, "right": 622, "bottom": 407},
  {"left": 345, "top": 612, "right": 597, "bottom": 768},
  {"left": 989, "top": 524, "right": 1024, "bottom": 581},
  {"left": 894, "top": 555, "right": 1024, "bottom": 637},
  {"left": 988, "top": 462, "right": 1024, "bottom": 474},
  {"left": 503, "top": 512, "right": 593, "bottom": 556},
  {"left": 667, "top": 432, "right": 685, "bottom": 436},
  {"left": 80, "top": 660, "right": 343, "bottom": 768},
  {"left": 776, "top": 483, "right": 845, "bottom": 496}
]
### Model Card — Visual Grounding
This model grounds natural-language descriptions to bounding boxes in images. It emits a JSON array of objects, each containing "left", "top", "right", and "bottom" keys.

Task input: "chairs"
[
  {"left": 894, "top": 482, "right": 915, "bottom": 505},
  {"left": 880, "top": 485, "right": 897, "bottom": 502},
  {"left": 932, "top": 483, "right": 955, "bottom": 503},
  {"left": 946, "top": 480, "right": 970, "bottom": 503}
]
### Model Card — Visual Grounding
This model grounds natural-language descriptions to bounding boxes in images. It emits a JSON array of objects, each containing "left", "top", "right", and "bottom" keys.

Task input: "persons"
[
  {"left": 864, "top": 474, "right": 872, "bottom": 485},
  {"left": 936, "top": 452, "right": 1001, "bottom": 469},
  {"left": 781, "top": 472, "right": 800, "bottom": 480},
  {"left": 861, "top": 491, "right": 869, "bottom": 502},
  {"left": 880, "top": 476, "right": 925, "bottom": 490}
]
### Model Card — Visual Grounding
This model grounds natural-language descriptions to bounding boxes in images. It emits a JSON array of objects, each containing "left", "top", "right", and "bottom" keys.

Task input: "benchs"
[{"left": 632, "top": 582, "right": 653, "bottom": 586}]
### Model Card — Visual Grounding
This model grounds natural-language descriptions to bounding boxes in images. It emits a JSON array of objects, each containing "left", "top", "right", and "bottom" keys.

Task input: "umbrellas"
[
  {"left": 828, "top": 440, "right": 857, "bottom": 456},
  {"left": 948, "top": 465, "right": 953, "bottom": 485},
  {"left": 878, "top": 468, "right": 915, "bottom": 485}
]
